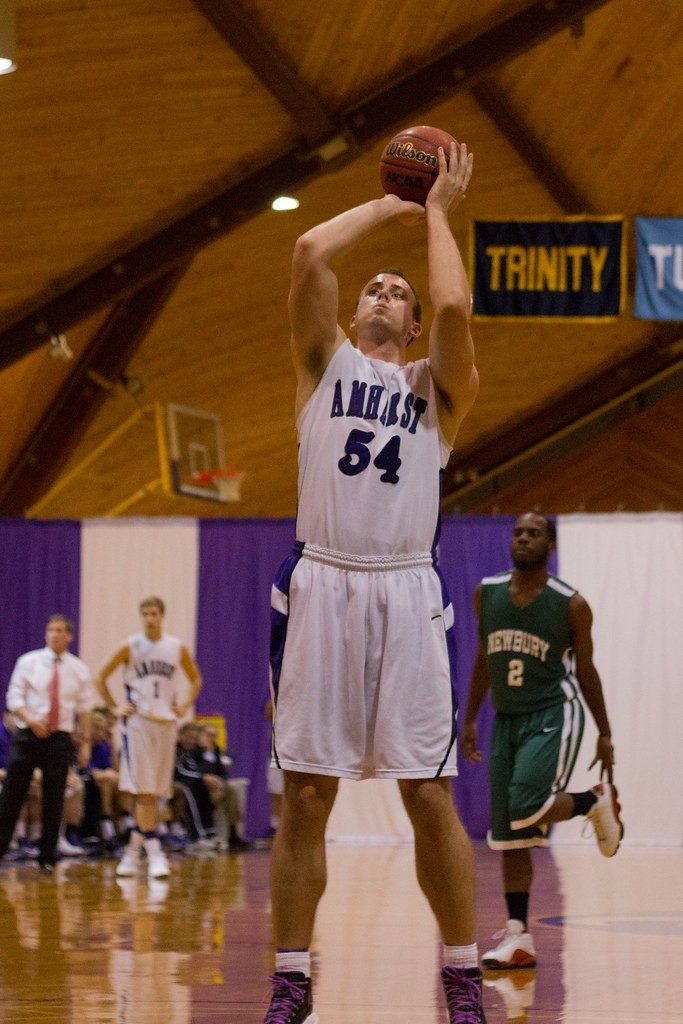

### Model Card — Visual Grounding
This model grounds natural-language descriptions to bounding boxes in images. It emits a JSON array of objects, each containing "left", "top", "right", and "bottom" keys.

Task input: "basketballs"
[{"left": 379, "top": 125, "right": 462, "bottom": 208}]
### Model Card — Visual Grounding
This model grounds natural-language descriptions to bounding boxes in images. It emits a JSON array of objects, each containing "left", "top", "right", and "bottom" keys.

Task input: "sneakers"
[
  {"left": 261, "top": 971, "right": 319, "bottom": 1024},
  {"left": 482, "top": 919, "right": 536, "bottom": 967},
  {"left": 580, "top": 781, "right": 624, "bottom": 858},
  {"left": 441, "top": 963, "right": 486, "bottom": 1024}
]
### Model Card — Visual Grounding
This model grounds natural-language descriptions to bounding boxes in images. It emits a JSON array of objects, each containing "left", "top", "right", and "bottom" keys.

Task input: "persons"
[
  {"left": 1, "top": 685, "right": 284, "bottom": 867},
  {"left": 262, "top": 142, "right": 492, "bottom": 1024},
  {"left": 0, "top": 614, "right": 98, "bottom": 874},
  {"left": 459, "top": 511, "right": 625, "bottom": 970},
  {"left": 98, "top": 595, "right": 204, "bottom": 879}
]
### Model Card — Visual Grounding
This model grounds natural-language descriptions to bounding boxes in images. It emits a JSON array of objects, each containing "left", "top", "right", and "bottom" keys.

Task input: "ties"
[{"left": 47, "top": 664, "right": 59, "bottom": 732}]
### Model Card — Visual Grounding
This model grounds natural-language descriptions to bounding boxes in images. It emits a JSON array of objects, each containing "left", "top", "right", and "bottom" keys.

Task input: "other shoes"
[
  {"left": 115, "top": 852, "right": 141, "bottom": 877},
  {"left": 145, "top": 851, "right": 170, "bottom": 877}
]
